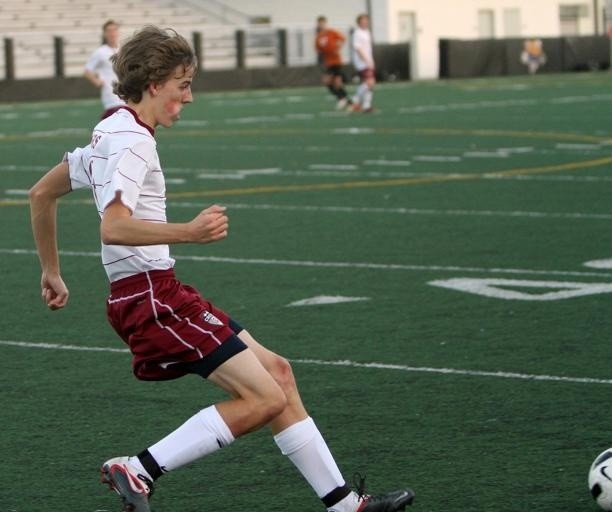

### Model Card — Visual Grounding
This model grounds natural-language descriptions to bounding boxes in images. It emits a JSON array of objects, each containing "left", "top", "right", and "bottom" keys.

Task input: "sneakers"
[
  {"left": 100, "top": 456, "right": 155, "bottom": 512},
  {"left": 327, "top": 472, "right": 414, "bottom": 512}
]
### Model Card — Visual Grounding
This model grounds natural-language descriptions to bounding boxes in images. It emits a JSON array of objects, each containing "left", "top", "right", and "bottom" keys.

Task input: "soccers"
[{"left": 588, "top": 447, "right": 612, "bottom": 512}]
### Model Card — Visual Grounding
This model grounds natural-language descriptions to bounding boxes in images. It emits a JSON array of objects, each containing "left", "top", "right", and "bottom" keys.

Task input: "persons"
[
  {"left": 81, "top": 19, "right": 121, "bottom": 111},
  {"left": 316, "top": 14, "right": 376, "bottom": 112},
  {"left": 29, "top": 26, "right": 417, "bottom": 512}
]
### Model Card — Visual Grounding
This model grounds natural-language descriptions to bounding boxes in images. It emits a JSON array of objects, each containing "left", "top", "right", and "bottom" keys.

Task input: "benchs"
[{"left": 1, "top": 1, "right": 276, "bottom": 81}]
